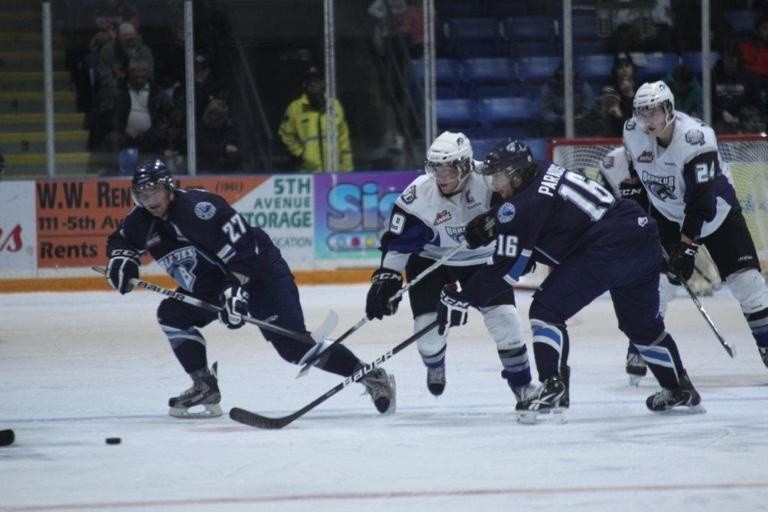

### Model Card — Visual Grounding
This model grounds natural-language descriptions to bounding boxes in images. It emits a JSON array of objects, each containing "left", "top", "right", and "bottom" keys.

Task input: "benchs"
[{"left": 4, "top": 3, "right": 169, "bottom": 179}]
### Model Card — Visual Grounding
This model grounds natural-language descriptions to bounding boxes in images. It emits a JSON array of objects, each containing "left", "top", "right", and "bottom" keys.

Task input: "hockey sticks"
[
  {"left": 659, "top": 244, "right": 736, "bottom": 359},
  {"left": 92, "top": 265, "right": 339, "bottom": 343},
  {"left": 296, "top": 217, "right": 497, "bottom": 376},
  {"left": 228, "top": 321, "right": 441, "bottom": 429}
]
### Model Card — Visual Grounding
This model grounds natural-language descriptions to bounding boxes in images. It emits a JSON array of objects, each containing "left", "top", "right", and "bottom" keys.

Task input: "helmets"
[
  {"left": 129, "top": 160, "right": 175, "bottom": 208},
  {"left": 632, "top": 80, "right": 675, "bottom": 126},
  {"left": 423, "top": 131, "right": 474, "bottom": 185},
  {"left": 480, "top": 138, "right": 535, "bottom": 179}
]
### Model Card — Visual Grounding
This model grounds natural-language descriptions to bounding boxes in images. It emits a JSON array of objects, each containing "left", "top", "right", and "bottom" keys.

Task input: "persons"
[
  {"left": 101, "top": 154, "right": 399, "bottom": 420},
  {"left": 621, "top": 74, "right": 767, "bottom": 388},
  {"left": 593, "top": 141, "right": 653, "bottom": 213},
  {"left": 434, "top": 134, "right": 707, "bottom": 416},
  {"left": 51, "top": 1, "right": 436, "bottom": 178},
  {"left": 536, "top": 0, "right": 765, "bottom": 138},
  {"left": 361, "top": 128, "right": 544, "bottom": 405}
]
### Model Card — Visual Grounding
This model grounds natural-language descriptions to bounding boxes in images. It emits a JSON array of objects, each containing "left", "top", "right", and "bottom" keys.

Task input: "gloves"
[
  {"left": 217, "top": 286, "right": 250, "bottom": 329},
  {"left": 106, "top": 249, "right": 141, "bottom": 293},
  {"left": 367, "top": 268, "right": 403, "bottom": 320},
  {"left": 436, "top": 283, "right": 471, "bottom": 335},
  {"left": 669, "top": 241, "right": 697, "bottom": 285}
]
